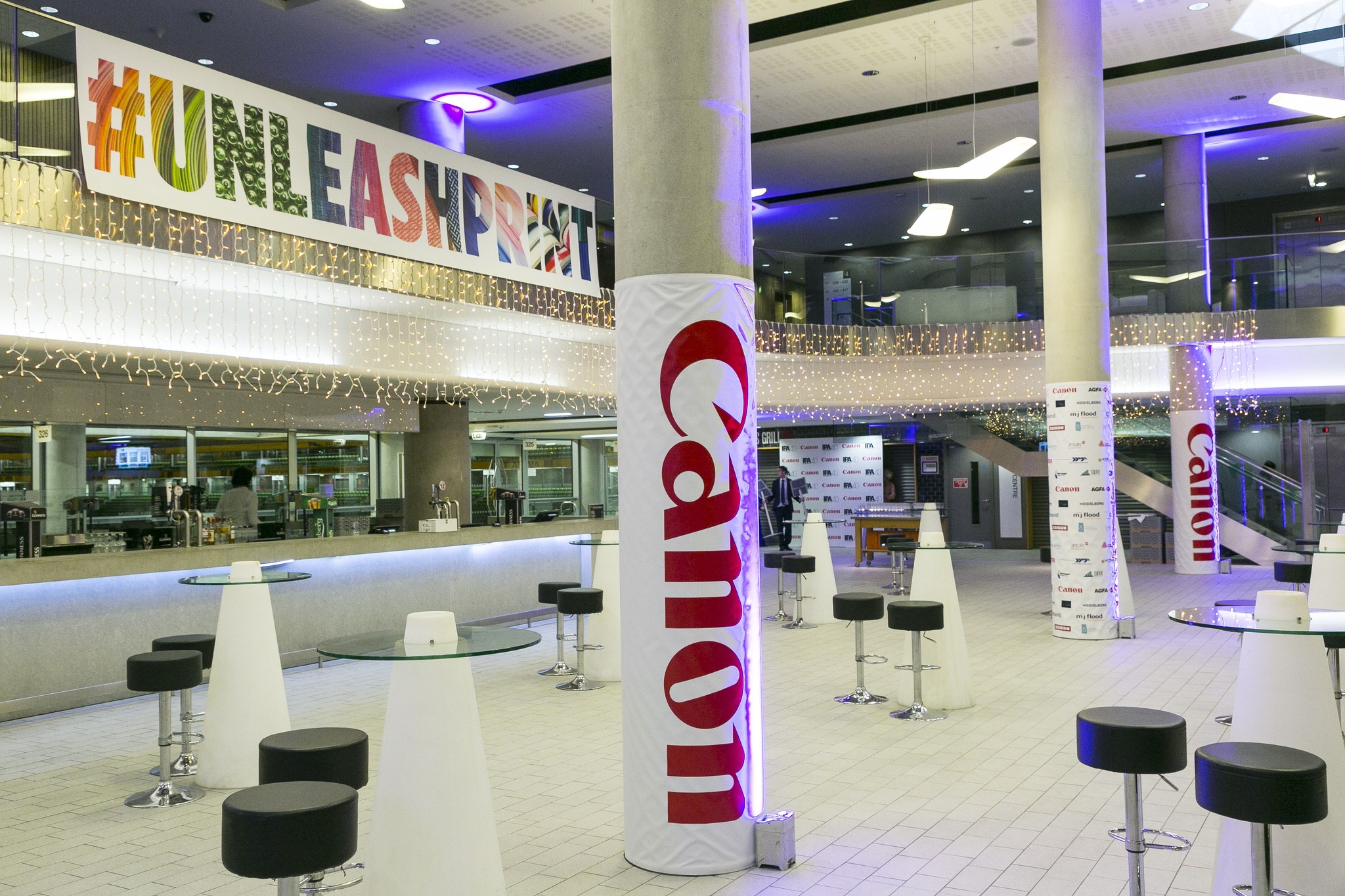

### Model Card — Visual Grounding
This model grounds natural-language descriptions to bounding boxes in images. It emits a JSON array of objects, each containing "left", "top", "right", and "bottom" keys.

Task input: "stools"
[
  {"left": 1195, "top": 742, "right": 1330, "bottom": 896},
  {"left": 150, "top": 629, "right": 219, "bottom": 776},
  {"left": 554, "top": 585, "right": 607, "bottom": 691},
  {"left": 832, "top": 591, "right": 889, "bottom": 706},
  {"left": 885, "top": 599, "right": 950, "bottom": 724},
  {"left": 257, "top": 727, "right": 366, "bottom": 896},
  {"left": 219, "top": 780, "right": 359, "bottom": 896},
  {"left": 887, "top": 535, "right": 914, "bottom": 597},
  {"left": 878, "top": 532, "right": 910, "bottom": 590},
  {"left": 1212, "top": 597, "right": 1257, "bottom": 727},
  {"left": 124, "top": 649, "right": 207, "bottom": 809},
  {"left": 1273, "top": 559, "right": 1314, "bottom": 593},
  {"left": 779, "top": 553, "right": 819, "bottom": 629},
  {"left": 537, "top": 581, "right": 579, "bottom": 676},
  {"left": 762, "top": 550, "right": 797, "bottom": 621},
  {"left": 1076, "top": 707, "right": 1191, "bottom": 896}
]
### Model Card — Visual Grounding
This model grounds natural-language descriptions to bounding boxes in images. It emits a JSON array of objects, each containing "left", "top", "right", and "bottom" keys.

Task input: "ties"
[{"left": 781, "top": 479, "right": 786, "bottom": 504}]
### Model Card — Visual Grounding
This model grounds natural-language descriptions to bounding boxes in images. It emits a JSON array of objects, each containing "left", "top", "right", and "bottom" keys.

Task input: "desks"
[
  {"left": 781, "top": 511, "right": 845, "bottom": 625},
  {"left": 568, "top": 530, "right": 622, "bottom": 682},
  {"left": 179, "top": 560, "right": 313, "bottom": 787},
  {"left": 879, "top": 501, "right": 985, "bottom": 710},
  {"left": 1167, "top": 510, "right": 1345, "bottom": 896},
  {"left": 316, "top": 610, "right": 542, "bottom": 896}
]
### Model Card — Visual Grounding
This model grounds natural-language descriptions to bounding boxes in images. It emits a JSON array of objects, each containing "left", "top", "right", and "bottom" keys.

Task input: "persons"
[
  {"left": 1255, "top": 460, "right": 1282, "bottom": 529},
  {"left": 758, "top": 479, "right": 772, "bottom": 547},
  {"left": 762, "top": 466, "right": 805, "bottom": 551},
  {"left": 883, "top": 468, "right": 896, "bottom": 503},
  {"left": 215, "top": 467, "right": 264, "bottom": 536}
]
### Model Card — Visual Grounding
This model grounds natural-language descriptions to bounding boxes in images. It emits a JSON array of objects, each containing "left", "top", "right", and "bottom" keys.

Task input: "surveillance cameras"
[{"left": 198, "top": 12, "right": 213, "bottom": 23}]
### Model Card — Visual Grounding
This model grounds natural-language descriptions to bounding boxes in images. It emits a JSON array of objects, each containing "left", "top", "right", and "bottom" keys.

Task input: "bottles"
[
  {"left": 228, "top": 518, "right": 235, "bottom": 544},
  {"left": 215, "top": 517, "right": 222, "bottom": 545},
  {"left": 208, "top": 517, "right": 215, "bottom": 545},
  {"left": 222, "top": 517, "right": 228, "bottom": 544},
  {"left": 201, "top": 515, "right": 209, "bottom": 545}
]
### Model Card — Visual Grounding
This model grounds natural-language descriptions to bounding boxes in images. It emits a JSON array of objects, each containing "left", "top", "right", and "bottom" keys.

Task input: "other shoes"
[
  {"left": 780, "top": 547, "right": 784, "bottom": 551},
  {"left": 784, "top": 546, "right": 793, "bottom": 550}
]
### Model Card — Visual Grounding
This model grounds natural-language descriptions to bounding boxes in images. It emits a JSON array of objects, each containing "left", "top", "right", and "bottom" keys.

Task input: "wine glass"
[
  {"left": 94, "top": 532, "right": 104, "bottom": 553},
  {"left": 116, "top": 532, "right": 127, "bottom": 552},
  {"left": 108, "top": 532, "right": 118, "bottom": 552},
  {"left": 235, "top": 525, "right": 250, "bottom": 528},
  {"left": 102, "top": 532, "right": 110, "bottom": 553}
]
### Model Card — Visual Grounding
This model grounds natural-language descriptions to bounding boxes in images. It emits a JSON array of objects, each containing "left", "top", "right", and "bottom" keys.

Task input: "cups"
[
  {"left": 235, "top": 528, "right": 258, "bottom": 543},
  {"left": 275, "top": 495, "right": 281, "bottom": 502},
  {"left": 307, "top": 500, "right": 311, "bottom": 509},
  {"left": 7, "top": 509, "right": 24, "bottom": 519},
  {"left": 502, "top": 492, "right": 514, "bottom": 498},
  {"left": 311, "top": 498, "right": 318, "bottom": 509},
  {"left": 352, "top": 521, "right": 359, "bottom": 535},
  {"left": 318, "top": 500, "right": 321, "bottom": 509}
]
